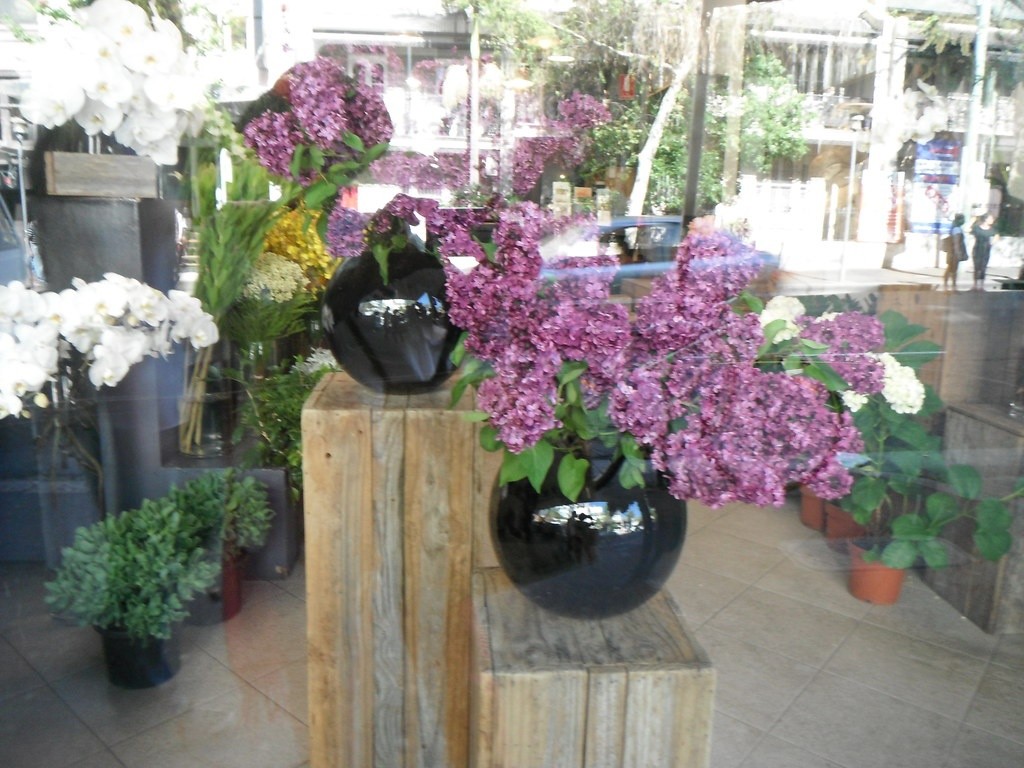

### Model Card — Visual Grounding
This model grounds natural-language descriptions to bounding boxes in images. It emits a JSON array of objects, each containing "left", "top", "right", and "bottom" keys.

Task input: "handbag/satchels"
[{"left": 941, "top": 233, "right": 953, "bottom": 252}]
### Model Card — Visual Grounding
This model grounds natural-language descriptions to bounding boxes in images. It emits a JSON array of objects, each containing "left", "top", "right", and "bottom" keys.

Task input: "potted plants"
[
  {"left": 840, "top": 311, "right": 1023, "bottom": 606},
  {"left": 44, "top": 467, "right": 277, "bottom": 687}
]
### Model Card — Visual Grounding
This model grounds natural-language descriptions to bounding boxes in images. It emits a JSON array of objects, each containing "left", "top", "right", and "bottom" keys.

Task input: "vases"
[
  {"left": 800, "top": 485, "right": 879, "bottom": 545},
  {"left": 178, "top": 336, "right": 233, "bottom": 459},
  {"left": 488, "top": 432, "right": 688, "bottom": 618},
  {"left": 322, "top": 249, "right": 466, "bottom": 395},
  {"left": 29, "top": 400, "right": 105, "bottom": 580}
]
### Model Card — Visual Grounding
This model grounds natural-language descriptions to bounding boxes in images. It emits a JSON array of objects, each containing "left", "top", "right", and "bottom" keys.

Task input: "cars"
[{"left": 523, "top": 218, "right": 780, "bottom": 303}]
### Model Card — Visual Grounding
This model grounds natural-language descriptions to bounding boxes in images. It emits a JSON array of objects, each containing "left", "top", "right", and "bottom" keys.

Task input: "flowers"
[{"left": 242, "top": 50, "right": 925, "bottom": 506}]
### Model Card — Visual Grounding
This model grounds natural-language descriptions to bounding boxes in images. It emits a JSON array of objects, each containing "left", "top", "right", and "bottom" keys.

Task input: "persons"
[
  {"left": 969, "top": 214, "right": 999, "bottom": 290},
  {"left": 566, "top": 511, "right": 596, "bottom": 566},
  {"left": 371, "top": 302, "right": 426, "bottom": 345},
  {"left": 944, "top": 214, "right": 968, "bottom": 290}
]
